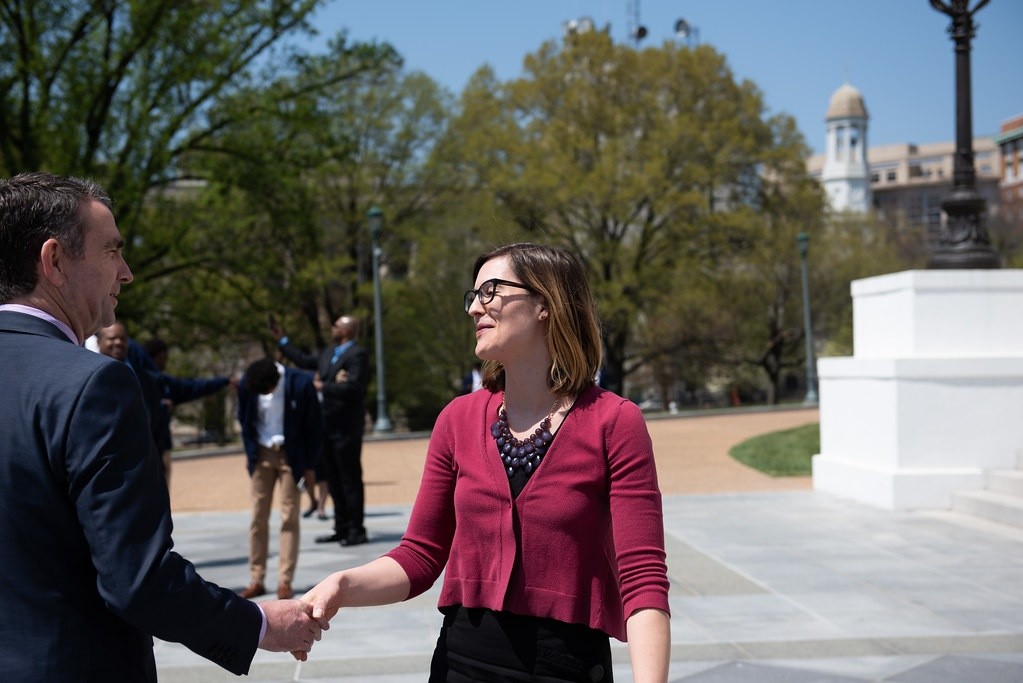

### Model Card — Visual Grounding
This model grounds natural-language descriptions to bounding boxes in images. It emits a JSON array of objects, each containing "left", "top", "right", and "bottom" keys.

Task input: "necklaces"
[{"left": 490, "top": 390, "right": 561, "bottom": 476}]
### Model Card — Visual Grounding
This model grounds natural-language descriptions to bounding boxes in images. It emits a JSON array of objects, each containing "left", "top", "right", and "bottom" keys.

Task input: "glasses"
[{"left": 464, "top": 278, "right": 541, "bottom": 313}]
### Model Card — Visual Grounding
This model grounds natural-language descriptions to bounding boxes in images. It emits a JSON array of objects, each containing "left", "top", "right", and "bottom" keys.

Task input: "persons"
[
  {"left": 0, "top": 173, "right": 329, "bottom": 683},
  {"left": 291, "top": 242, "right": 671, "bottom": 683},
  {"left": 83, "top": 320, "right": 236, "bottom": 489},
  {"left": 236, "top": 359, "right": 319, "bottom": 603},
  {"left": 270, "top": 314, "right": 370, "bottom": 547}
]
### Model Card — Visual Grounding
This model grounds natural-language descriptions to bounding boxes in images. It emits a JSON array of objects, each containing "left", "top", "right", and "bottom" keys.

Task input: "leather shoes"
[
  {"left": 240, "top": 583, "right": 265, "bottom": 599},
  {"left": 278, "top": 585, "right": 292, "bottom": 599}
]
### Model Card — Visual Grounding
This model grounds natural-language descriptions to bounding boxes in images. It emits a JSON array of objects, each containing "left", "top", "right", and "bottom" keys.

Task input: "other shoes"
[
  {"left": 303, "top": 501, "right": 318, "bottom": 517},
  {"left": 318, "top": 514, "right": 327, "bottom": 520},
  {"left": 316, "top": 532, "right": 347, "bottom": 543},
  {"left": 341, "top": 531, "right": 366, "bottom": 546}
]
